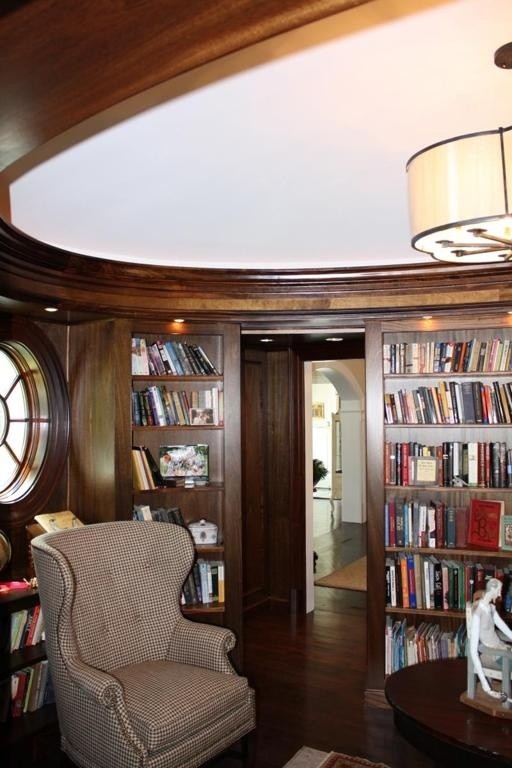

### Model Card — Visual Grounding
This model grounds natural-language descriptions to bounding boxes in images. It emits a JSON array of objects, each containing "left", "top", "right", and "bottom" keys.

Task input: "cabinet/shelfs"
[
  {"left": 0, "top": 587, "right": 59, "bottom": 746},
  {"left": 68, "top": 316, "right": 243, "bottom": 672},
  {"left": 363, "top": 315, "right": 512, "bottom": 709}
]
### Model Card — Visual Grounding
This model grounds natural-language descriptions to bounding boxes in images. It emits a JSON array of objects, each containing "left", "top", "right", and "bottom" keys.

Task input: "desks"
[{"left": 384, "top": 653, "right": 512, "bottom": 767}]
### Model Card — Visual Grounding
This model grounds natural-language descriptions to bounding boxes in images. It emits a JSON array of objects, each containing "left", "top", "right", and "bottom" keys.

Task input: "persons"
[
  {"left": 506, "top": 524, "right": 510, "bottom": 540},
  {"left": 468, "top": 575, "right": 512, "bottom": 708}
]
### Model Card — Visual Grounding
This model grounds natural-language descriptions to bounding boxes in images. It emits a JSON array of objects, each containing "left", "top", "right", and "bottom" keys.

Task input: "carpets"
[
  {"left": 283, "top": 743, "right": 387, "bottom": 767},
  {"left": 313, "top": 555, "right": 368, "bottom": 594}
]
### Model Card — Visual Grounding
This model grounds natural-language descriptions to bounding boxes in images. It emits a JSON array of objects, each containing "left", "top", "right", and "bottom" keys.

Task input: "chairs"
[{"left": 32, "top": 518, "right": 258, "bottom": 768}]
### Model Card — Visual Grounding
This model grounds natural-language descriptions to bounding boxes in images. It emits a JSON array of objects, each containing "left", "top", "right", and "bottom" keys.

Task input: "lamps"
[{"left": 404, "top": 39, "right": 512, "bottom": 267}]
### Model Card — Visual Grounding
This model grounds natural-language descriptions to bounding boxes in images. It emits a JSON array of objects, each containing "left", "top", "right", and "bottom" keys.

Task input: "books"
[
  {"left": 7, "top": 605, "right": 50, "bottom": 717},
  {"left": 384, "top": 496, "right": 505, "bottom": 548},
  {"left": 131, "top": 337, "right": 225, "bottom": 609},
  {"left": 34, "top": 511, "right": 85, "bottom": 533},
  {"left": 385, "top": 615, "right": 468, "bottom": 675},
  {"left": 385, "top": 552, "right": 511, "bottom": 612},
  {"left": 384, "top": 381, "right": 511, "bottom": 424},
  {"left": 383, "top": 441, "right": 511, "bottom": 488},
  {"left": 383, "top": 339, "right": 511, "bottom": 374}
]
